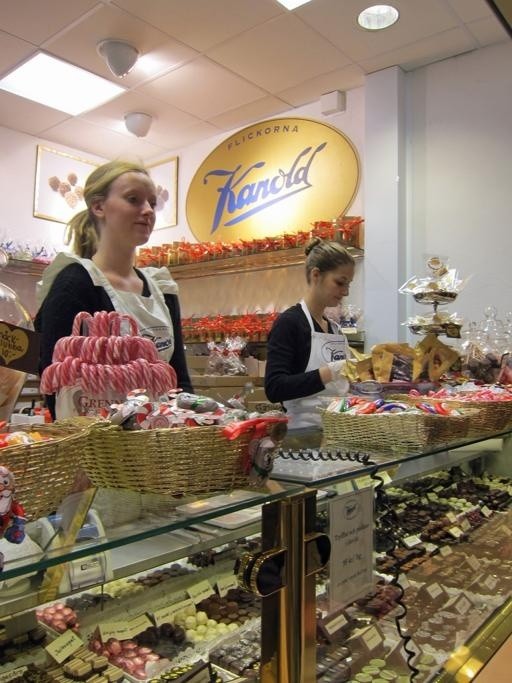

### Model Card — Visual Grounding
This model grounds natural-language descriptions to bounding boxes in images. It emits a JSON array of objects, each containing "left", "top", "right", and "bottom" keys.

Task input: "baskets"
[
  {"left": 0, "top": 416, "right": 284, "bottom": 525},
  {"left": 315, "top": 394, "right": 512, "bottom": 454}
]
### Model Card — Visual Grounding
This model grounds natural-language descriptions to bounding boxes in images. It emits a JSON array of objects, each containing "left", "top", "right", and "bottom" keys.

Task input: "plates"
[{"left": 176, "top": 488, "right": 280, "bottom": 529}]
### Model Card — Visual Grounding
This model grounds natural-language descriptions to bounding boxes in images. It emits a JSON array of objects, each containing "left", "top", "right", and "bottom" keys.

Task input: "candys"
[
  {"left": 0, "top": 311, "right": 284, "bottom": 448},
  {"left": 332, "top": 383, "right": 512, "bottom": 417}
]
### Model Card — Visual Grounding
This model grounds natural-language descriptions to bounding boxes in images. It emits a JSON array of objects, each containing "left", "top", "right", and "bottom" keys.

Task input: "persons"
[
  {"left": 265, "top": 237, "right": 358, "bottom": 431},
  {"left": 32, "top": 160, "right": 196, "bottom": 421}
]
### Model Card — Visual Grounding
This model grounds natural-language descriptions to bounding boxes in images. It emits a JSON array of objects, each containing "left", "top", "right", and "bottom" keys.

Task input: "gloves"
[{"left": 327, "top": 358, "right": 358, "bottom": 382}]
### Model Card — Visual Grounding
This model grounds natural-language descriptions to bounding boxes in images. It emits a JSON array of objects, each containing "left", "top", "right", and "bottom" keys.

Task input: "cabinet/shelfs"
[{"left": 0, "top": 424, "right": 511, "bottom": 683}]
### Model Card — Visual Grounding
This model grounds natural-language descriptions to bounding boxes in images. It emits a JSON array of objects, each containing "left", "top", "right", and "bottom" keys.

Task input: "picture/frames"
[{"left": 33, "top": 144, "right": 179, "bottom": 231}]
